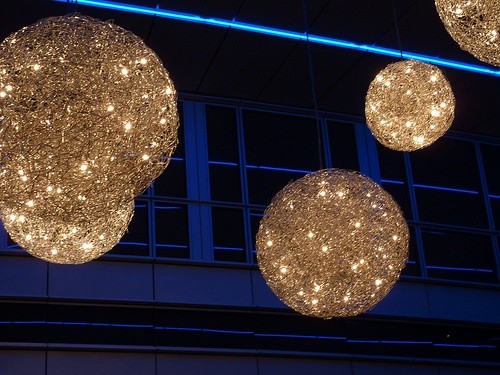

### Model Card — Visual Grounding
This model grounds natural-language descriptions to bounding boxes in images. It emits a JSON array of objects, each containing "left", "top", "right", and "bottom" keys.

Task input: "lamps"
[
  {"left": 434, "top": 0, "right": 500, "bottom": 67},
  {"left": 256, "top": 168, "right": 410, "bottom": 320},
  {"left": 364, "top": 57, "right": 455, "bottom": 151},
  {"left": 0, "top": 198, "right": 136, "bottom": 265},
  {"left": 1, "top": 14, "right": 181, "bottom": 224}
]
